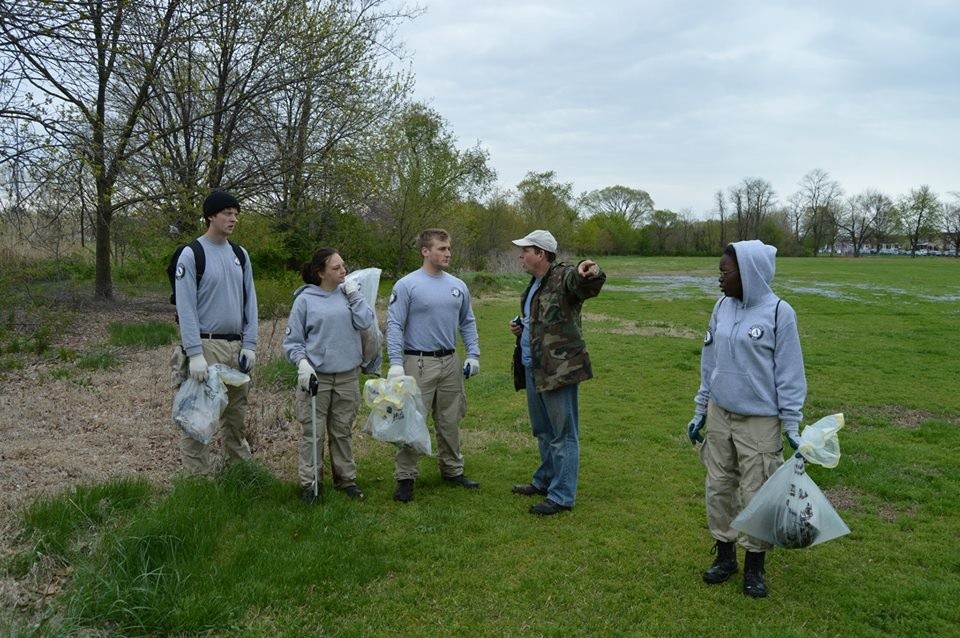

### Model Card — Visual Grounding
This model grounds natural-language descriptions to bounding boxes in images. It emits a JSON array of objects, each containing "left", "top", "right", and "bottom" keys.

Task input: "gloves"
[
  {"left": 297, "top": 359, "right": 320, "bottom": 392},
  {"left": 688, "top": 413, "right": 705, "bottom": 445},
  {"left": 238, "top": 349, "right": 256, "bottom": 374},
  {"left": 190, "top": 354, "right": 208, "bottom": 382},
  {"left": 387, "top": 366, "right": 405, "bottom": 379},
  {"left": 344, "top": 278, "right": 360, "bottom": 295},
  {"left": 786, "top": 430, "right": 807, "bottom": 463},
  {"left": 463, "top": 359, "right": 480, "bottom": 379}
]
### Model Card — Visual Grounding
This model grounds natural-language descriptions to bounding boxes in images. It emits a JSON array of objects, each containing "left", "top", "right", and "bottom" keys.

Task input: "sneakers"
[
  {"left": 530, "top": 499, "right": 572, "bottom": 515},
  {"left": 512, "top": 485, "right": 548, "bottom": 496}
]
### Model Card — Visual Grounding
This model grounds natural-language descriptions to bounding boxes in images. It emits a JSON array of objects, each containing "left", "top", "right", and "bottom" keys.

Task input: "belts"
[
  {"left": 201, "top": 334, "right": 242, "bottom": 342},
  {"left": 404, "top": 349, "right": 455, "bottom": 357}
]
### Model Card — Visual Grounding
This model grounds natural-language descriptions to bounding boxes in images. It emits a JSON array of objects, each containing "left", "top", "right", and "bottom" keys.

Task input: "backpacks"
[{"left": 170, "top": 239, "right": 247, "bottom": 325}]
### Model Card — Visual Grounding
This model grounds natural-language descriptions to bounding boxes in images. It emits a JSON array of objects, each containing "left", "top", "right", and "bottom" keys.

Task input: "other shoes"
[
  {"left": 344, "top": 486, "right": 364, "bottom": 500},
  {"left": 443, "top": 475, "right": 479, "bottom": 488},
  {"left": 302, "top": 483, "right": 322, "bottom": 504},
  {"left": 394, "top": 479, "right": 414, "bottom": 503}
]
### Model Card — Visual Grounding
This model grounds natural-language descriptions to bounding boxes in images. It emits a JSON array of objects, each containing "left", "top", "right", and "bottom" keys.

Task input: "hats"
[
  {"left": 203, "top": 191, "right": 239, "bottom": 218},
  {"left": 512, "top": 230, "right": 558, "bottom": 254}
]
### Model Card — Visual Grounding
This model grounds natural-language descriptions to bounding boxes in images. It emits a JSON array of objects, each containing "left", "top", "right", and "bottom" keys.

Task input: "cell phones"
[{"left": 513, "top": 316, "right": 524, "bottom": 332}]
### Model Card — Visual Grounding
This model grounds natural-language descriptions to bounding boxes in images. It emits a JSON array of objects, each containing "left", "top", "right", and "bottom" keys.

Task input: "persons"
[
  {"left": 169, "top": 191, "right": 258, "bottom": 479},
  {"left": 509, "top": 230, "right": 606, "bottom": 516},
  {"left": 386, "top": 229, "right": 480, "bottom": 504},
  {"left": 282, "top": 247, "right": 374, "bottom": 507},
  {"left": 688, "top": 240, "right": 806, "bottom": 598}
]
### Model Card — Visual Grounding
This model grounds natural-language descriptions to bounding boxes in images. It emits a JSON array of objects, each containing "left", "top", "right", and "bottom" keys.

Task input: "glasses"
[{"left": 719, "top": 270, "right": 737, "bottom": 278}]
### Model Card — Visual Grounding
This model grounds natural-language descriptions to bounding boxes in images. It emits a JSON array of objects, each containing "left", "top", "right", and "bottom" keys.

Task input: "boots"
[
  {"left": 744, "top": 551, "right": 768, "bottom": 597},
  {"left": 703, "top": 541, "right": 738, "bottom": 583}
]
